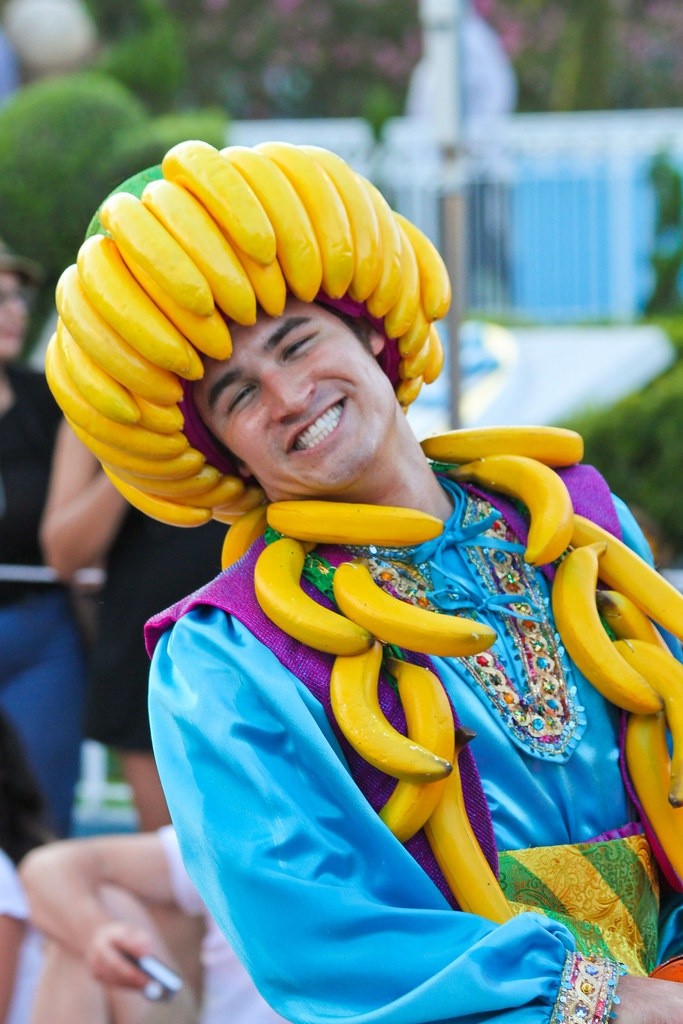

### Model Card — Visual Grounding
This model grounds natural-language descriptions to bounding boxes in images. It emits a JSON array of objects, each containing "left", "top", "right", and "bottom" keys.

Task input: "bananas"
[{"left": 45, "top": 140, "right": 683, "bottom": 929}]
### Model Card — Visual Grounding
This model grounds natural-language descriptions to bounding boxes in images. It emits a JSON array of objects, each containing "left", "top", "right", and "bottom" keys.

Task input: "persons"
[
  {"left": 44, "top": 135, "right": 683, "bottom": 1024},
  {"left": 1, "top": 246, "right": 299, "bottom": 1024}
]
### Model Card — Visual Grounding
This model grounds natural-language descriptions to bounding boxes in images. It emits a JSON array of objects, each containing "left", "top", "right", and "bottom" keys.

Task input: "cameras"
[{"left": 123, "top": 948, "right": 183, "bottom": 1004}]
